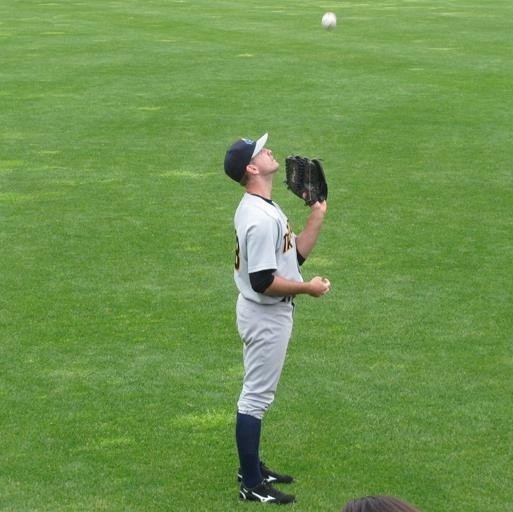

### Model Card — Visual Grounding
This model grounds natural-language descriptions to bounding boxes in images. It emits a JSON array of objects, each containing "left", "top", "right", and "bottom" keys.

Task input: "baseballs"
[{"left": 322, "top": 12, "right": 336, "bottom": 29}]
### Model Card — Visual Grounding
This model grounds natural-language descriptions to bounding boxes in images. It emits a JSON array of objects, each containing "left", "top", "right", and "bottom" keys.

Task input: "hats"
[{"left": 224, "top": 130, "right": 269, "bottom": 183}]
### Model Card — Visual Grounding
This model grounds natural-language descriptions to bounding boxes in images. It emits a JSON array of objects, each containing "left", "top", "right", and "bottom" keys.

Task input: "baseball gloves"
[{"left": 284, "top": 153, "right": 327, "bottom": 206}]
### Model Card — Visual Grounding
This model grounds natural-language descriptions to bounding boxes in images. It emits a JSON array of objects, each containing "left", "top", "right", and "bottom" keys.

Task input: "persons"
[{"left": 219, "top": 133, "right": 330, "bottom": 504}]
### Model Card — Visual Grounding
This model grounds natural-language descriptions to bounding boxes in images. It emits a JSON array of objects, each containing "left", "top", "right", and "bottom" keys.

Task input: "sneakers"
[
  {"left": 239, "top": 481, "right": 295, "bottom": 504},
  {"left": 238, "top": 462, "right": 295, "bottom": 485}
]
